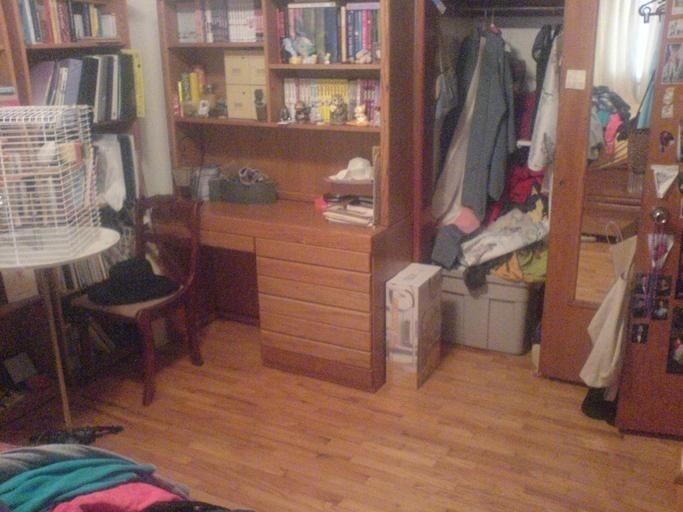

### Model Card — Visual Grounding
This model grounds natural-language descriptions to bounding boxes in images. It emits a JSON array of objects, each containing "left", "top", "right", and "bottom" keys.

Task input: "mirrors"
[{"left": 575, "top": 0, "right": 663, "bottom": 312}]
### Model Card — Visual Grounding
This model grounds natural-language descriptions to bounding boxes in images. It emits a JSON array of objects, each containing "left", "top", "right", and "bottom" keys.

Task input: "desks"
[
  {"left": 136, "top": 187, "right": 412, "bottom": 394},
  {"left": 1, "top": 224, "right": 127, "bottom": 448}
]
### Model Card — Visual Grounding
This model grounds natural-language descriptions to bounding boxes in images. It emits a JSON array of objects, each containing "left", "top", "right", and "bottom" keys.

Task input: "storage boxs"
[
  {"left": 427, "top": 259, "right": 543, "bottom": 356},
  {"left": 384, "top": 262, "right": 444, "bottom": 391},
  {"left": 224, "top": 47, "right": 268, "bottom": 120}
]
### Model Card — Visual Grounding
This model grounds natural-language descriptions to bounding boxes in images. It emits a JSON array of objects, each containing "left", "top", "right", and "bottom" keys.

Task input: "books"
[
  {"left": 175, "top": 0, "right": 380, "bottom": 125},
  {"left": 323, "top": 201, "right": 375, "bottom": 228}
]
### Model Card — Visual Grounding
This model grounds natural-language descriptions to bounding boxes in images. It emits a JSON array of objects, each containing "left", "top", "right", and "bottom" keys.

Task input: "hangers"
[
  {"left": 434, "top": 4, "right": 564, "bottom": 35},
  {"left": 637, "top": 0, "right": 664, "bottom": 23}
]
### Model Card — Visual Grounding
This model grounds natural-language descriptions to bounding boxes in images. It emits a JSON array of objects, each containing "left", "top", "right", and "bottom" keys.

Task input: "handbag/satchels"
[{"left": 578, "top": 220, "right": 629, "bottom": 390}]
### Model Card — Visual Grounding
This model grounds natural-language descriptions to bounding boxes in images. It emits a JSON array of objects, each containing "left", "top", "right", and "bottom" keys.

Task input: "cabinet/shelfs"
[
  {"left": 6, "top": 0, "right": 134, "bottom": 389},
  {"left": 537, "top": 2, "right": 663, "bottom": 391},
  {"left": 158, "top": 0, "right": 413, "bottom": 229}
]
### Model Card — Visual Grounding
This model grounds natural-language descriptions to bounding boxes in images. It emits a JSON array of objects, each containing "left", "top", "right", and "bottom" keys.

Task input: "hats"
[{"left": 87, "top": 259, "right": 177, "bottom": 306}]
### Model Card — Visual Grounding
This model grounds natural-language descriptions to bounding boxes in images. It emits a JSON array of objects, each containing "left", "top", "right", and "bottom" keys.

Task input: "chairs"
[{"left": 62, "top": 196, "right": 204, "bottom": 405}]
